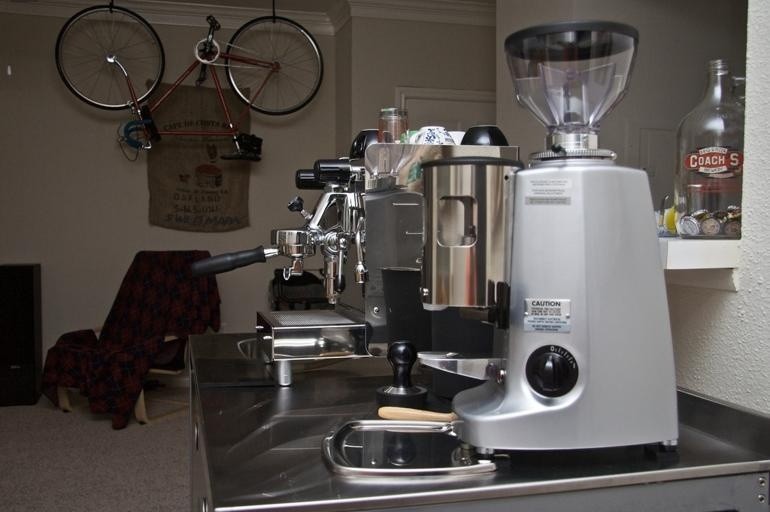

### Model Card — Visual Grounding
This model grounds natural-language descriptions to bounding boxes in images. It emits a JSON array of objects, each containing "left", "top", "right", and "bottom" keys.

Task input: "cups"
[{"left": 409, "top": 125, "right": 457, "bottom": 146}]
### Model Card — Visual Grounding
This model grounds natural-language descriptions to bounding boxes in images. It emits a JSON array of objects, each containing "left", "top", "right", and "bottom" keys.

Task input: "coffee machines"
[{"left": 376, "top": 16, "right": 681, "bottom": 465}]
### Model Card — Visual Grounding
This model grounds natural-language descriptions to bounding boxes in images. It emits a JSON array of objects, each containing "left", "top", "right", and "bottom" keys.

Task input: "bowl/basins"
[{"left": 459, "top": 125, "right": 509, "bottom": 147}]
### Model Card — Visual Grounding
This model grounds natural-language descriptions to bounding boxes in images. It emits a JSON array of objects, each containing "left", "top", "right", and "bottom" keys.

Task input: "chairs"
[{"left": 44, "top": 249, "right": 217, "bottom": 426}]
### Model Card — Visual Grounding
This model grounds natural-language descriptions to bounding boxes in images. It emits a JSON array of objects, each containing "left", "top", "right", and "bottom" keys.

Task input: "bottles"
[
  {"left": 376, "top": 107, "right": 407, "bottom": 145},
  {"left": 670, "top": 58, "right": 745, "bottom": 242}
]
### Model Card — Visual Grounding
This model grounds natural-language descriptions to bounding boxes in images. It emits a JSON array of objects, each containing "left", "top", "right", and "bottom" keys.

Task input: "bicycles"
[{"left": 51, "top": 1, "right": 326, "bottom": 164}]
